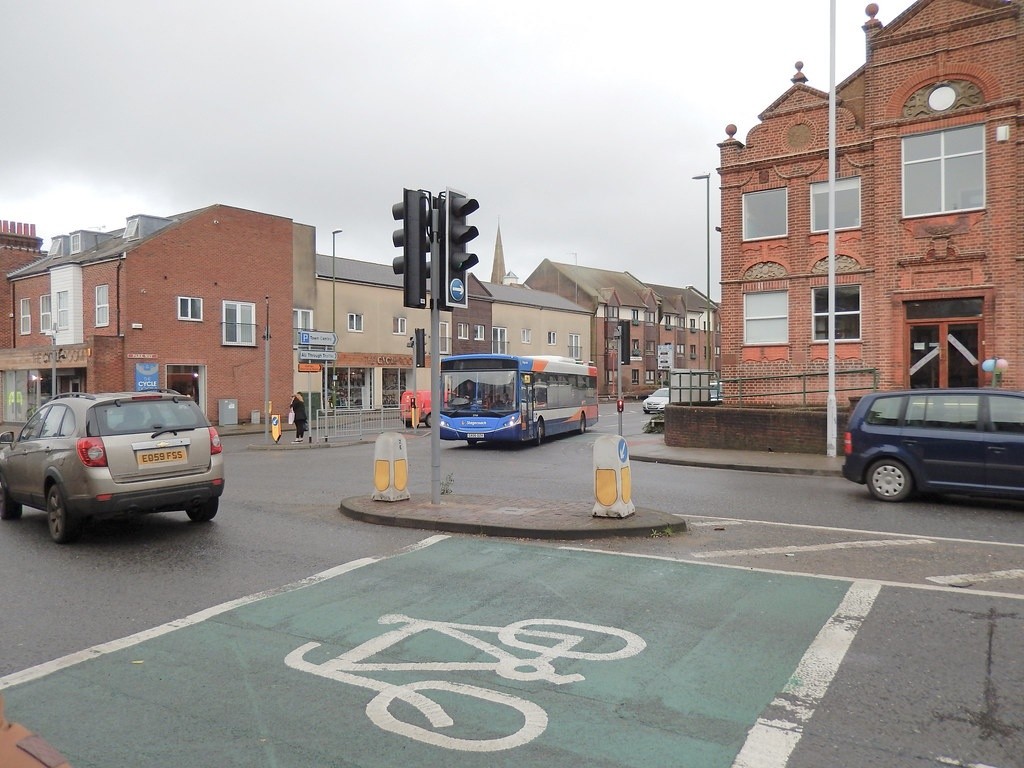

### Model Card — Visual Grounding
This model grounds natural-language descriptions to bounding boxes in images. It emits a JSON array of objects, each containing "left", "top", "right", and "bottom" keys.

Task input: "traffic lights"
[
  {"left": 392, "top": 188, "right": 426, "bottom": 310},
  {"left": 444, "top": 187, "right": 479, "bottom": 309}
]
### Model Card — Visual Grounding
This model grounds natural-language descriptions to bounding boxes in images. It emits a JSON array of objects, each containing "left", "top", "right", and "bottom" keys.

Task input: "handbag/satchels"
[{"left": 288, "top": 407, "right": 295, "bottom": 424}]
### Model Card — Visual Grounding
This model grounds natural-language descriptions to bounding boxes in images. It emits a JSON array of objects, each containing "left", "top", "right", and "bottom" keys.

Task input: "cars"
[
  {"left": 840, "top": 386, "right": 1024, "bottom": 504},
  {"left": 710, "top": 383, "right": 723, "bottom": 401},
  {"left": 642, "top": 387, "right": 670, "bottom": 413}
]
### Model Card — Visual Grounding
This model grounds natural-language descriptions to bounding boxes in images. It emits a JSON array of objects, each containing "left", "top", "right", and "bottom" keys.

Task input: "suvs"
[{"left": 0, "top": 390, "right": 225, "bottom": 544}]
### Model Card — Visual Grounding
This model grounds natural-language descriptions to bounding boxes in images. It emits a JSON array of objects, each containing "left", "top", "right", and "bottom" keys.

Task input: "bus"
[{"left": 439, "top": 356, "right": 599, "bottom": 445}]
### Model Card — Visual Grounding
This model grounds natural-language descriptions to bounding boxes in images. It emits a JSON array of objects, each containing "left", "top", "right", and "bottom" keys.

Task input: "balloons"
[{"left": 982, "top": 358, "right": 1008, "bottom": 375}]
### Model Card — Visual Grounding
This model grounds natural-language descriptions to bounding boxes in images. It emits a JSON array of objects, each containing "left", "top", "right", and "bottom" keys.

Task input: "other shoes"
[{"left": 294, "top": 438, "right": 304, "bottom": 442}]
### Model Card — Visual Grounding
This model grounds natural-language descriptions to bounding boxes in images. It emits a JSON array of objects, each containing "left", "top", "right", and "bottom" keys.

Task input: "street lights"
[
  {"left": 40, "top": 330, "right": 57, "bottom": 397},
  {"left": 332, "top": 230, "right": 343, "bottom": 409},
  {"left": 692, "top": 174, "right": 712, "bottom": 372}
]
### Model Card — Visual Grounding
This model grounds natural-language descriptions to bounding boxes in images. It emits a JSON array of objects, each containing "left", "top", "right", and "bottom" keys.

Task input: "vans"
[{"left": 400, "top": 391, "right": 458, "bottom": 428}]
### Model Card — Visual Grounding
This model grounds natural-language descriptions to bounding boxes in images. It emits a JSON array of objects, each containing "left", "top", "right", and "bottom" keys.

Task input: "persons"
[
  {"left": 9, "top": 390, "right": 23, "bottom": 422},
  {"left": 289, "top": 392, "right": 307, "bottom": 444},
  {"left": 491, "top": 392, "right": 509, "bottom": 410},
  {"left": 459, "top": 380, "right": 475, "bottom": 399}
]
